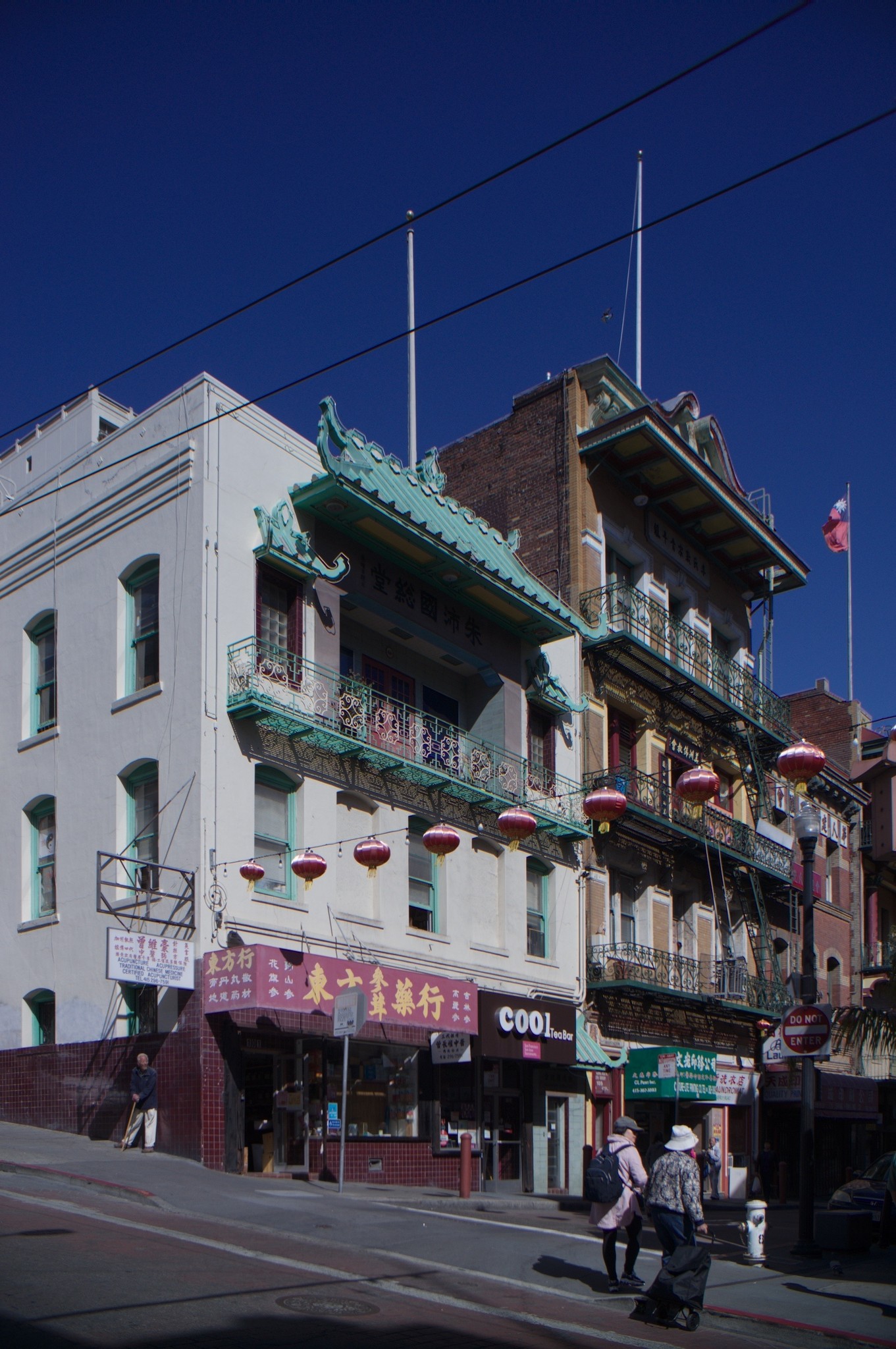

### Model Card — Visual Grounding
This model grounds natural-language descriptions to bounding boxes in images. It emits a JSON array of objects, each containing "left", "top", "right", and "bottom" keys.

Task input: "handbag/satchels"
[
  {"left": 702, "top": 1162, "right": 709, "bottom": 1178},
  {"left": 752, "top": 1177, "right": 762, "bottom": 1193}
]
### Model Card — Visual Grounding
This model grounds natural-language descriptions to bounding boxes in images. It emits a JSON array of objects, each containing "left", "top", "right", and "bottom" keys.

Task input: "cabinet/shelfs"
[
  {"left": 327, "top": 1053, "right": 389, "bottom": 1097},
  {"left": 245, "top": 1054, "right": 273, "bottom": 1144}
]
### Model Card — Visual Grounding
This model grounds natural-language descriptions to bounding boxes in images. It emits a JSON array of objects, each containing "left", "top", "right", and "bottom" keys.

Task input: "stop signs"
[{"left": 780, "top": 1003, "right": 834, "bottom": 1056}]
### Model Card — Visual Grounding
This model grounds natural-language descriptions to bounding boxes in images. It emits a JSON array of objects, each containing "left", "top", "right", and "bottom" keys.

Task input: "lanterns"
[{"left": 232, "top": 739, "right": 825, "bottom": 891}]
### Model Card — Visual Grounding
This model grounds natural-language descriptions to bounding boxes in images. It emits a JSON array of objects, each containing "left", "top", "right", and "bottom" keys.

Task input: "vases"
[{"left": 594, "top": 775, "right": 616, "bottom": 791}]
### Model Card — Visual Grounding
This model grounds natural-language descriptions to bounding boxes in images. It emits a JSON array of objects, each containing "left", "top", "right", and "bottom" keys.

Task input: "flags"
[{"left": 821, "top": 496, "right": 851, "bottom": 552}]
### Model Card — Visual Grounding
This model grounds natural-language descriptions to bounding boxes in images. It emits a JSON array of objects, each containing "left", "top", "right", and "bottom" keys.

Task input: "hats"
[
  {"left": 664, "top": 1124, "right": 699, "bottom": 1151},
  {"left": 613, "top": 1116, "right": 645, "bottom": 1133}
]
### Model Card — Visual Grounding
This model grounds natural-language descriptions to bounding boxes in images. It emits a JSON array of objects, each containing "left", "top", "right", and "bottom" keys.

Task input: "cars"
[{"left": 827, "top": 1148, "right": 896, "bottom": 1244}]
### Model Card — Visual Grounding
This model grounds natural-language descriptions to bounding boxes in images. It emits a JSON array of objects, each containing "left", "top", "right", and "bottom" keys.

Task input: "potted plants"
[
  {"left": 335, "top": 666, "right": 375, "bottom": 740},
  {"left": 467, "top": 740, "right": 508, "bottom": 789}
]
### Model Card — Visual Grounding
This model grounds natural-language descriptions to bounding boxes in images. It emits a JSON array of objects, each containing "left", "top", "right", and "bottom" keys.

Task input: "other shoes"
[
  {"left": 114, "top": 1142, "right": 130, "bottom": 1149},
  {"left": 142, "top": 1147, "right": 153, "bottom": 1153},
  {"left": 710, "top": 1196, "right": 719, "bottom": 1200},
  {"left": 765, "top": 1200, "right": 770, "bottom": 1204}
]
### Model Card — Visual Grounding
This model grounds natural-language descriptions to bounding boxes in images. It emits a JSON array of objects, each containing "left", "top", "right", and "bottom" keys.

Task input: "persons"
[
  {"left": 112, "top": 1052, "right": 159, "bottom": 1153},
  {"left": 753, "top": 1141, "right": 778, "bottom": 1204},
  {"left": 644, "top": 1124, "right": 707, "bottom": 1263},
  {"left": 703, "top": 1137, "right": 723, "bottom": 1199},
  {"left": 588, "top": 1114, "right": 654, "bottom": 1290}
]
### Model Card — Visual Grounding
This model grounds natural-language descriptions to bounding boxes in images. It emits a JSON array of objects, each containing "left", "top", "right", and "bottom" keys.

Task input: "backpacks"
[{"left": 585, "top": 1142, "right": 635, "bottom": 1203}]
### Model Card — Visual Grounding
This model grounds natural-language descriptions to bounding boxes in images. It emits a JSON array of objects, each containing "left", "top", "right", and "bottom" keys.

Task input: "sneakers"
[
  {"left": 620, "top": 1272, "right": 645, "bottom": 1286},
  {"left": 608, "top": 1278, "right": 619, "bottom": 1292}
]
